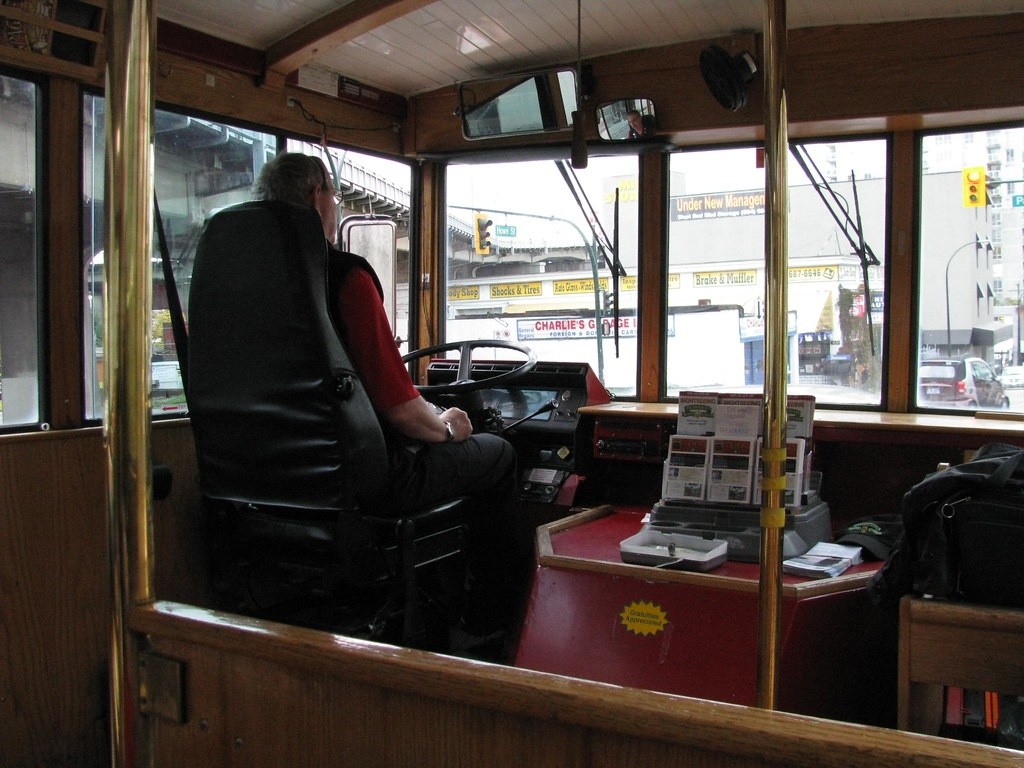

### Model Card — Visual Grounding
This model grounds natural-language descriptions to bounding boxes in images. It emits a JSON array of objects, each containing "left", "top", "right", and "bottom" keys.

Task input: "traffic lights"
[
  {"left": 473, "top": 213, "right": 492, "bottom": 254},
  {"left": 962, "top": 167, "right": 985, "bottom": 209}
]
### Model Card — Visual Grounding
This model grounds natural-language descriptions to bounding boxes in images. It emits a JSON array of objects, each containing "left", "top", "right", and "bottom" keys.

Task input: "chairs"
[{"left": 185, "top": 201, "right": 476, "bottom": 657}]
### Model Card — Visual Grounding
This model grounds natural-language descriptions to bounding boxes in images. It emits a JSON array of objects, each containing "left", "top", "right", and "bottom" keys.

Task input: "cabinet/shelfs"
[{"left": 896, "top": 594, "right": 1023, "bottom": 748}]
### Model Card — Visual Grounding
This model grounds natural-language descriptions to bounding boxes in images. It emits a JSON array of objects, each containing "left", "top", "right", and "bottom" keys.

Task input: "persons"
[
  {"left": 253, "top": 153, "right": 531, "bottom": 633},
  {"left": 627, "top": 110, "right": 649, "bottom": 138}
]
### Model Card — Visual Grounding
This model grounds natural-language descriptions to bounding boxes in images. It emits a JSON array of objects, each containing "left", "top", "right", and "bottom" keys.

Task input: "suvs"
[{"left": 918, "top": 356, "right": 1010, "bottom": 409}]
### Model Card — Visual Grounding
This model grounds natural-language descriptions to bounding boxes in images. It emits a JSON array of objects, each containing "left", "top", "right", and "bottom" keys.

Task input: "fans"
[{"left": 700, "top": 45, "right": 758, "bottom": 112}]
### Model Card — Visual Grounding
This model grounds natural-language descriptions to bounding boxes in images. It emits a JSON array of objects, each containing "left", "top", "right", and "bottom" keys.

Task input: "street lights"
[{"left": 946, "top": 240, "right": 990, "bottom": 357}]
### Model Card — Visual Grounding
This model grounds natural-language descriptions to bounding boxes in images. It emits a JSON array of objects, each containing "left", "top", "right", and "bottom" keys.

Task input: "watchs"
[{"left": 444, "top": 420, "right": 454, "bottom": 440}]
